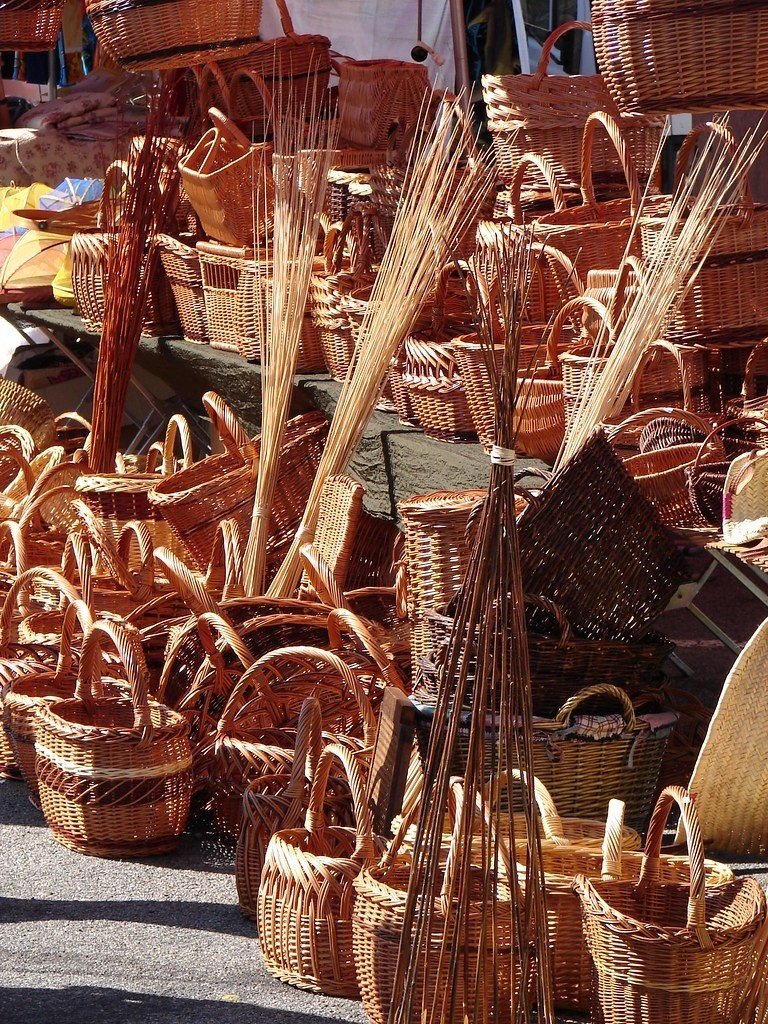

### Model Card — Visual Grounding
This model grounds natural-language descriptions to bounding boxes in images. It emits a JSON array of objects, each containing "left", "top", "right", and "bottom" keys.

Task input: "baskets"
[{"left": 0, "top": 0, "right": 768, "bottom": 1024}]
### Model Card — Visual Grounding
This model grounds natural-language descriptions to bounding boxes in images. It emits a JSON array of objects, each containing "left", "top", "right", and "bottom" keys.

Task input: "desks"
[{"left": 0, "top": 302, "right": 554, "bottom": 528}]
